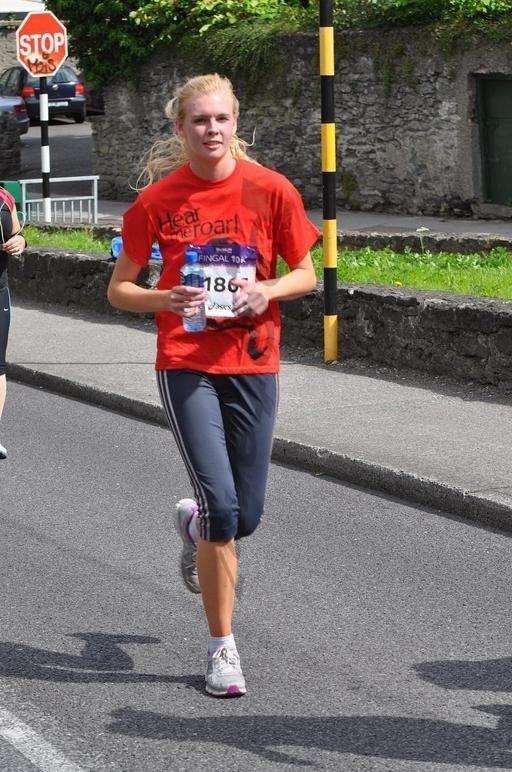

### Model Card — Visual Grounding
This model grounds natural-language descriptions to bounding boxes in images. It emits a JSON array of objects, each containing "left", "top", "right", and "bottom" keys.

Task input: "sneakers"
[
  {"left": 174, "top": 499, "right": 203, "bottom": 594},
  {"left": 206, "top": 646, "right": 247, "bottom": 697}
]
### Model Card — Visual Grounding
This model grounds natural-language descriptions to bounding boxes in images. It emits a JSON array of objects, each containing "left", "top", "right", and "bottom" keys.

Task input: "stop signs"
[{"left": 17, "top": 11, "right": 69, "bottom": 77}]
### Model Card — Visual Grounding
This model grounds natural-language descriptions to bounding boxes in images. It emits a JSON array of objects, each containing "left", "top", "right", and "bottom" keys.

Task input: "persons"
[
  {"left": 1, "top": 181, "right": 31, "bottom": 459},
  {"left": 107, "top": 72, "right": 324, "bottom": 700}
]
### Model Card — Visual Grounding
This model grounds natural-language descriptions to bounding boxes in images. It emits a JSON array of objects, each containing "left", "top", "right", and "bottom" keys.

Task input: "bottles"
[
  {"left": 145, "top": 240, "right": 164, "bottom": 287},
  {"left": 179, "top": 251, "right": 206, "bottom": 332}
]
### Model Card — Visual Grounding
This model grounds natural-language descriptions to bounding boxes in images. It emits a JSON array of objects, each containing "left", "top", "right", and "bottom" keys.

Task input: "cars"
[{"left": 0, "top": 64, "right": 87, "bottom": 136}]
